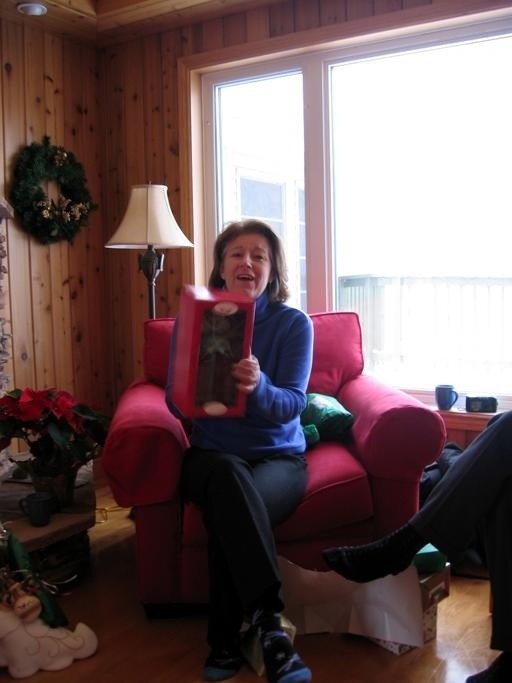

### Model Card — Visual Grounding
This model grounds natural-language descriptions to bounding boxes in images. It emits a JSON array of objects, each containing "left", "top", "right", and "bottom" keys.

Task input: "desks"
[{"left": 0, "top": 511, "right": 96, "bottom": 586}]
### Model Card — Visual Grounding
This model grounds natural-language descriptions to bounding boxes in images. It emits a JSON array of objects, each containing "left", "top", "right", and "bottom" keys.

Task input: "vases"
[{"left": 30, "top": 474, "right": 76, "bottom": 509}]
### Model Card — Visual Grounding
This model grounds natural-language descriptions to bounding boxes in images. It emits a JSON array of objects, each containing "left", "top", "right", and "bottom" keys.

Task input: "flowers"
[
  {"left": 7, "top": 134, "right": 98, "bottom": 245},
  {"left": 0, "top": 386, "right": 113, "bottom": 481}
]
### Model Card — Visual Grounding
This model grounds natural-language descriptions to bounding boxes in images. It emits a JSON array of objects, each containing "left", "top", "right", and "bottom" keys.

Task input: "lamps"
[{"left": 103, "top": 181, "right": 195, "bottom": 319}]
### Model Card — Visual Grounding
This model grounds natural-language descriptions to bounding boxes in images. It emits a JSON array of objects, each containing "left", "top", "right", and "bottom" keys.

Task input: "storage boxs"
[{"left": 167, "top": 286, "right": 257, "bottom": 421}]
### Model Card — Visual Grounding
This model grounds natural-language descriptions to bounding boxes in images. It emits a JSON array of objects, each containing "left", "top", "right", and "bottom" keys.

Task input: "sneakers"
[
  {"left": 320, "top": 535, "right": 409, "bottom": 582},
  {"left": 466, "top": 650, "right": 511, "bottom": 683}
]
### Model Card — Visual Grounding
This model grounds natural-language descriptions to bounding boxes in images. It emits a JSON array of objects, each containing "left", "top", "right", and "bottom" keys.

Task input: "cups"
[{"left": 433, "top": 383, "right": 459, "bottom": 412}]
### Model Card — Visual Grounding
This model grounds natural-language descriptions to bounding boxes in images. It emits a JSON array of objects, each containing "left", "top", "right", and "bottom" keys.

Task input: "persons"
[
  {"left": 320, "top": 409, "right": 512, "bottom": 683},
  {"left": 164, "top": 218, "right": 312, "bottom": 682}
]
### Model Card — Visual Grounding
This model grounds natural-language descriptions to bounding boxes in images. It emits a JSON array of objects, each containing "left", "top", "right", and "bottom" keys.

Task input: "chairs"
[{"left": 101, "top": 311, "right": 447, "bottom": 624}]
